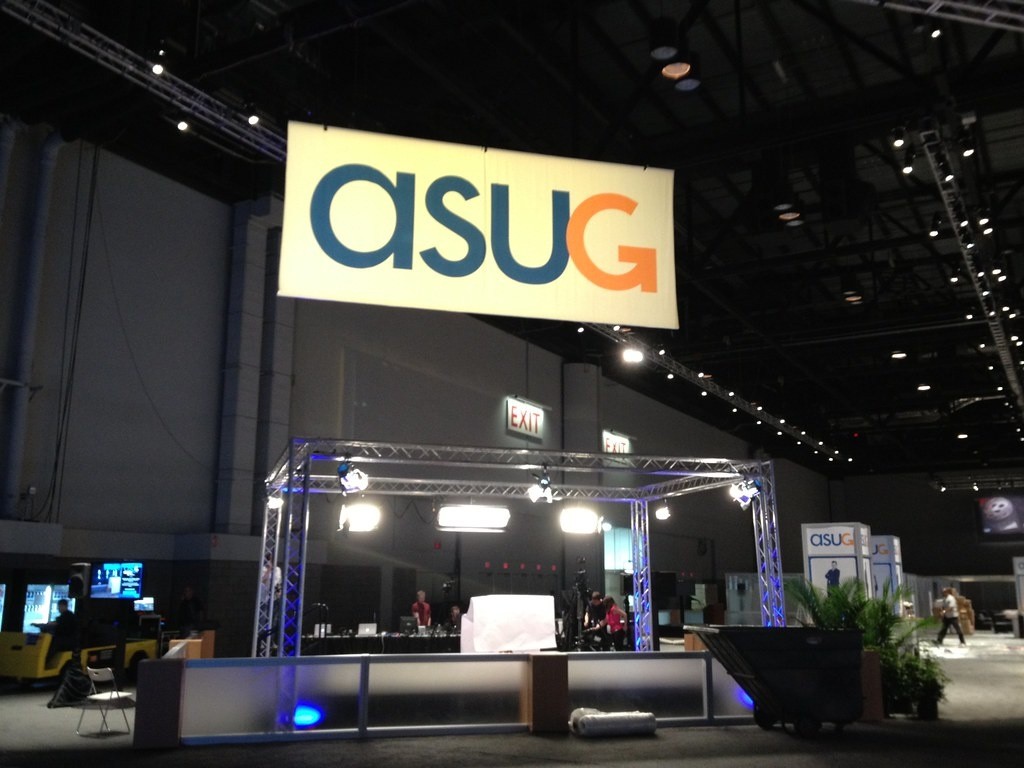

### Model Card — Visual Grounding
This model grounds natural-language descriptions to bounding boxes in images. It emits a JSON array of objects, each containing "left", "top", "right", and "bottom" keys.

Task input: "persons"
[
  {"left": 176, "top": 585, "right": 204, "bottom": 628},
  {"left": 583, "top": 591, "right": 628, "bottom": 651},
  {"left": 443, "top": 605, "right": 461, "bottom": 630},
  {"left": 825, "top": 561, "right": 840, "bottom": 593},
  {"left": 932, "top": 588, "right": 965, "bottom": 646},
  {"left": 411, "top": 590, "right": 432, "bottom": 626},
  {"left": 32, "top": 599, "right": 82, "bottom": 660}
]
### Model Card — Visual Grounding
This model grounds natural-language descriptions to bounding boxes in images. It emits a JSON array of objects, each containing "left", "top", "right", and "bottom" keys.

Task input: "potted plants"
[{"left": 781, "top": 567, "right": 942, "bottom": 724}]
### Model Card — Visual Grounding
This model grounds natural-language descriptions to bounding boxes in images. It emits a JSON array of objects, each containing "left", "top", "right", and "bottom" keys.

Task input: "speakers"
[{"left": 68, "top": 563, "right": 91, "bottom": 599}]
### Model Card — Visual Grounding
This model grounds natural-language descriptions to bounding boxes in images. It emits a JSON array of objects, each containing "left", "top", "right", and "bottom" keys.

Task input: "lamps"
[
  {"left": 656, "top": 499, "right": 673, "bottom": 521},
  {"left": 911, "top": 15, "right": 941, "bottom": 40},
  {"left": 428, "top": 504, "right": 515, "bottom": 534},
  {"left": 524, "top": 466, "right": 560, "bottom": 506},
  {"left": 573, "top": 324, "right": 852, "bottom": 465},
  {"left": 723, "top": 470, "right": 761, "bottom": 508},
  {"left": 147, "top": 58, "right": 260, "bottom": 141},
  {"left": 888, "top": 122, "right": 1024, "bottom": 496},
  {"left": 333, "top": 460, "right": 372, "bottom": 495}
]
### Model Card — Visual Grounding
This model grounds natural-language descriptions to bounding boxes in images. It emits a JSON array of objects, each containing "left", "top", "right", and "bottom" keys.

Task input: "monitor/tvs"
[
  {"left": 399, "top": 616, "right": 418, "bottom": 635},
  {"left": 355, "top": 623, "right": 377, "bottom": 637},
  {"left": 90, "top": 560, "right": 145, "bottom": 599}
]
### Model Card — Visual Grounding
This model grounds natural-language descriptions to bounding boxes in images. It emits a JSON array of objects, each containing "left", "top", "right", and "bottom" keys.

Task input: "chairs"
[{"left": 72, "top": 667, "right": 137, "bottom": 738}]
